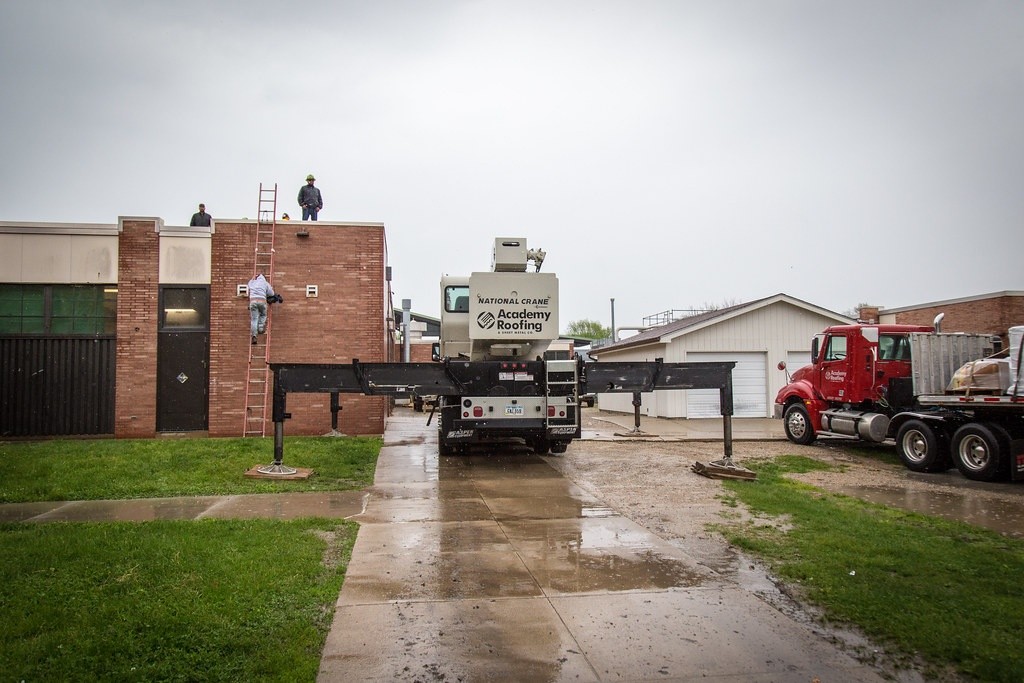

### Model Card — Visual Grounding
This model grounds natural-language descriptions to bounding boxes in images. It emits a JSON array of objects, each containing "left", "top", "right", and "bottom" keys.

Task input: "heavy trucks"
[{"left": 771, "top": 310, "right": 1024, "bottom": 483}]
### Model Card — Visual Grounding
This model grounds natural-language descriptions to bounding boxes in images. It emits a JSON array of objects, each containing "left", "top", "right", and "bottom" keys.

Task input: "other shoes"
[
  {"left": 251, "top": 337, "right": 257, "bottom": 344},
  {"left": 259, "top": 331, "right": 265, "bottom": 334}
]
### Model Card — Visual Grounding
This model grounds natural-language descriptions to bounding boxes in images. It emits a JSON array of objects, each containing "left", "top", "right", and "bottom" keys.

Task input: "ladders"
[{"left": 241, "top": 182, "right": 281, "bottom": 439}]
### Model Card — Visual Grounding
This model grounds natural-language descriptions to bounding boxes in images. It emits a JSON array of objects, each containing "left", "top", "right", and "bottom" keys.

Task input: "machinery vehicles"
[{"left": 257, "top": 235, "right": 747, "bottom": 477}]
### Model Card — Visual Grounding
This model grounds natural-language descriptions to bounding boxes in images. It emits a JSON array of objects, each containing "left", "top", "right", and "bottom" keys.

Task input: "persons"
[
  {"left": 297, "top": 174, "right": 324, "bottom": 221},
  {"left": 190, "top": 203, "right": 212, "bottom": 226},
  {"left": 248, "top": 274, "right": 275, "bottom": 345}
]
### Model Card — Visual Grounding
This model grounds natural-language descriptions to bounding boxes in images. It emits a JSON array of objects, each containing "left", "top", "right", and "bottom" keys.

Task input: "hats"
[
  {"left": 282, "top": 213, "right": 289, "bottom": 219},
  {"left": 306, "top": 175, "right": 315, "bottom": 181}
]
